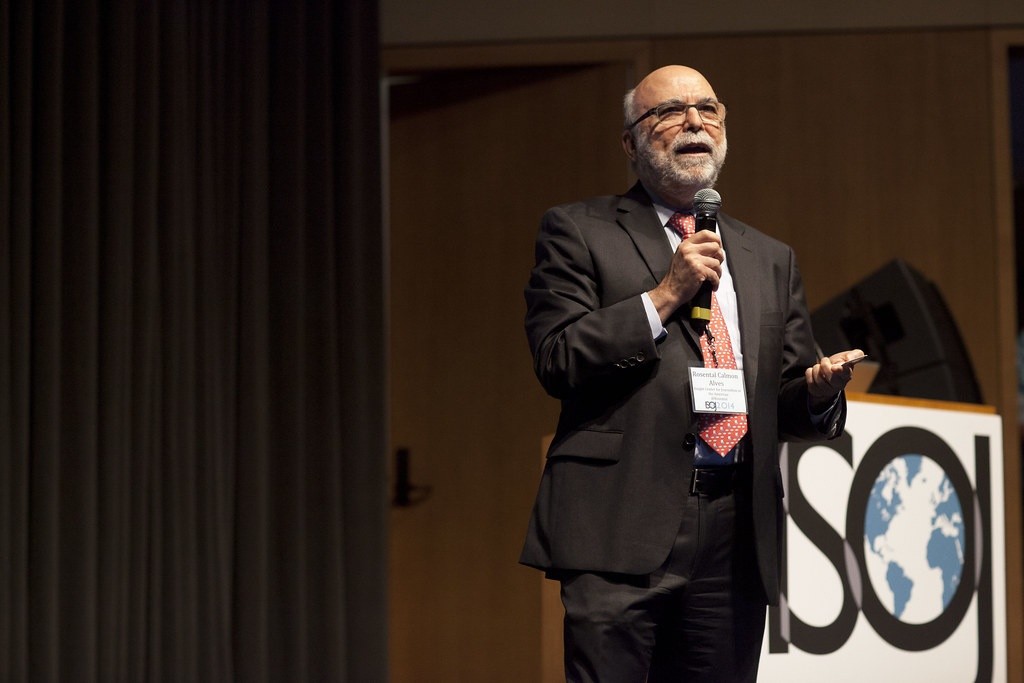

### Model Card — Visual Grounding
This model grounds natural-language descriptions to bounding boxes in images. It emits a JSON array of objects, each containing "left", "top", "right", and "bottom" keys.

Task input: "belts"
[{"left": 687, "top": 466, "right": 744, "bottom": 497}]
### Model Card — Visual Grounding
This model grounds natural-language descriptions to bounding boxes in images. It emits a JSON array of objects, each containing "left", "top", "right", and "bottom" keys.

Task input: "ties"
[{"left": 666, "top": 212, "right": 750, "bottom": 459}]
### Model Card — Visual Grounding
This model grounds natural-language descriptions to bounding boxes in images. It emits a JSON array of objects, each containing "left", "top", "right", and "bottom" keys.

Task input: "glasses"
[{"left": 628, "top": 99, "right": 721, "bottom": 132}]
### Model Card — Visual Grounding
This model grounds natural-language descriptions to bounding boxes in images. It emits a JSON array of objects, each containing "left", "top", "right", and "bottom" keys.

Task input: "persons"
[{"left": 519, "top": 65, "right": 865, "bottom": 683}]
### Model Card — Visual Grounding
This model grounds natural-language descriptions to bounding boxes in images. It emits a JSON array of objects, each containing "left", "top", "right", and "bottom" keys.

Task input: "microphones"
[{"left": 689, "top": 188, "right": 722, "bottom": 326}]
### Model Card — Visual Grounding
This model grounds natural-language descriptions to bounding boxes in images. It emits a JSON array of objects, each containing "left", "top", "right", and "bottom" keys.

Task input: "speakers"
[{"left": 808, "top": 259, "right": 984, "bottom": 404}]
